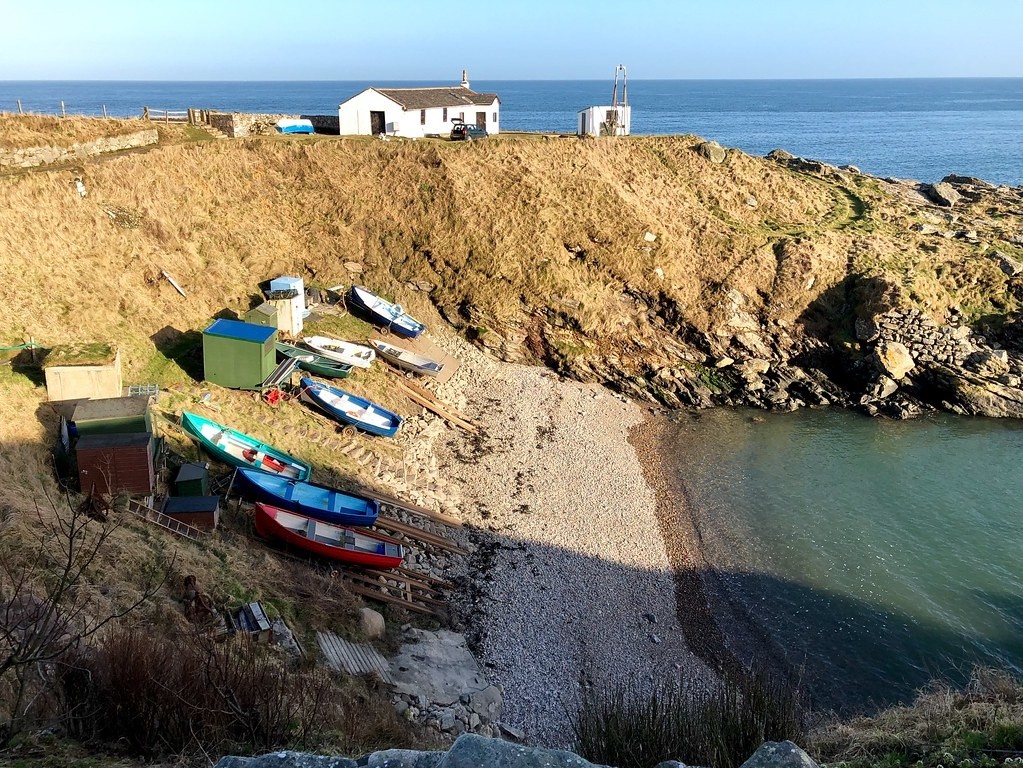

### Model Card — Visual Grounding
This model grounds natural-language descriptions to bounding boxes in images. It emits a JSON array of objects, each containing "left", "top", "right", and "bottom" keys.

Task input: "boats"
[
  {"left": 300, "top": 376, "right": 404, "bottom": 439},
  {"left": 180, "top": 408, "right": 311, "bottom": 483},
  {"left": 352, "top": 284, "right": 425, "bottom": 339},
  {"left": 303, "top": 336, "right": 376, "bottom": 369},
  {"left": 255, "top": 500, "right": 403, "bottom": 569},
  {"left": 274, "top": 341, "right": 353, "bottom": 379},
  {"left": 236, "top": 467, "right": 379, "bottom": 527},
  {"left": 366, "top": 335, "right": 444, "bottom": 377}
]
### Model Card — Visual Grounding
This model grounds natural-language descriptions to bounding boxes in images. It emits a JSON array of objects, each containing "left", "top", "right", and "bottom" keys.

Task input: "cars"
[{"left": 450, "top": 123, "right": 489, "bottom": 141}]
